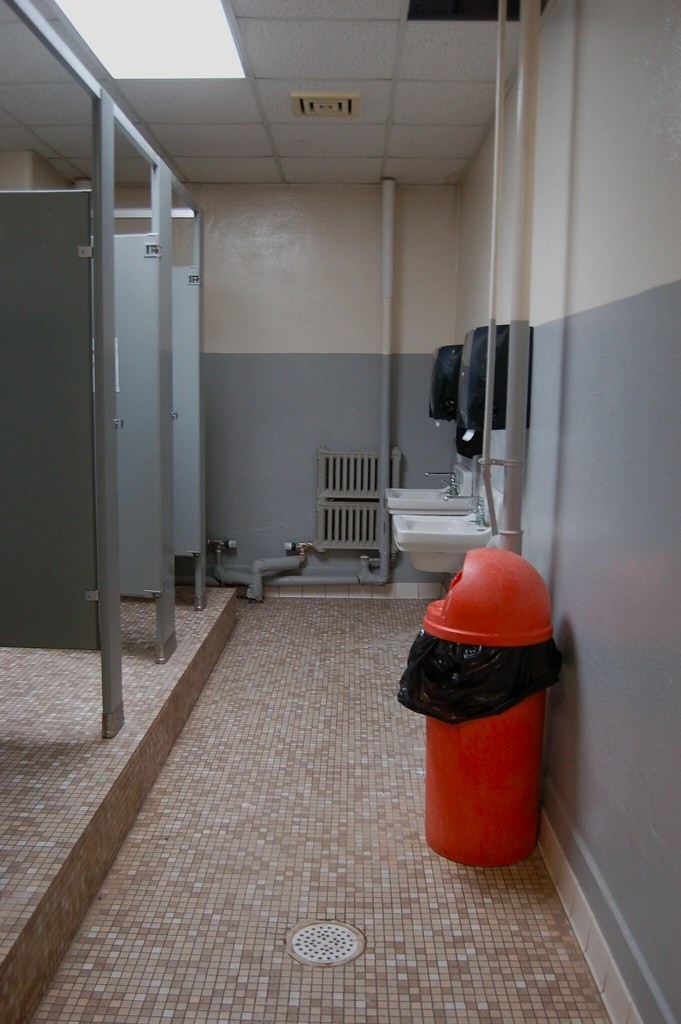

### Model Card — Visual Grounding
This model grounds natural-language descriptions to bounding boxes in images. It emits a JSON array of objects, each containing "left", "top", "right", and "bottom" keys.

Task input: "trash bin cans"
[{"left": 397, "top": 547, "right": 561, "bottom": 868}]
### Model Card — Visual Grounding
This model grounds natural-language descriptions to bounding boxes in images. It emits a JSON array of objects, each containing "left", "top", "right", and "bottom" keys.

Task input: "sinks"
[
  {"left": 393, "top": 513, "right": 491, "bottom": 553},
  {"left": 384, "top": 483, "right": 472, "bottom": 514}
]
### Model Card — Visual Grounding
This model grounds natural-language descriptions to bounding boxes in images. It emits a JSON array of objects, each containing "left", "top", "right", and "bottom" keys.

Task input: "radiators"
[{"left": 314, "top": 445, "right": 403, "bottom": 556}]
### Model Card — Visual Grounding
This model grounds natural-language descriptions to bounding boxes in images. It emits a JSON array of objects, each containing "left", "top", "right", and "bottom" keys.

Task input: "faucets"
[
  {"left": 442, "top": 493, "right": 486, "bottom": 526},
  {"left": 423, "top": 469, "right": 458, "bottom": 495}
]
